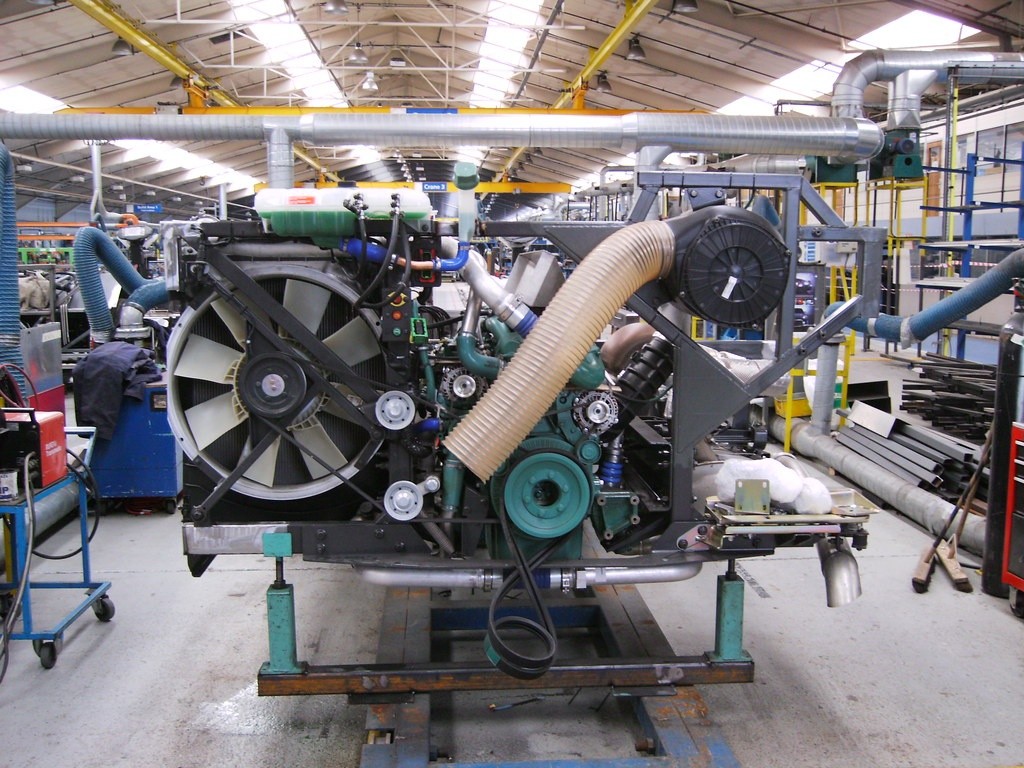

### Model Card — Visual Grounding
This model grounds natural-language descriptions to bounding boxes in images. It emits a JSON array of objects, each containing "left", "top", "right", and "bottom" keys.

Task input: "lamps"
[
  {"left": 169, "top": 77, "right": 182, "bottom": 89},
  {"left": 625, "top": 39, "right": 647, "bottom": 60},
  {"left": 517, "top": 162, "right": 526, "bottom": 171},
  {"left": 349, "top": 4, "right": 370, "bottom": 64},
  {"left": 390, "top": 58, "right": 407, "bottom": 67},
  {"left": 112, "top": 36, "right": 133, "bottom": 56},
  {"left": 406, "top": 177, "right": 413, "bottom": 182},
  {"left": 508, "top": 177, "right": 512, "bottom": 182},
  {"left": 400, "top": 163, "right": 411, "bottom": 171},
  {"left": 673, "top": 0, "right": 698, "bottom": 12},
  {"left": 533, "top": 147, "right": 543, "bottom": 156},
  {"left": 403, "top": 170, "right": 412, "bottom": 177},
  {"left": 392, "top": 147, "right": 403, "bottom": 158},
  {"left": 397, "top": 154, "right": 406, "bottom": 163},
  {"left": 524, "top": 154, "right": 533, "bottom": 164},
  {"left": 324, "top": 0, "right": 350, "bottom": 16},
  {"left": 595, "top": 75, "right": 612, "bottom": 91},
  {"left": 511, "top": 170, "right": 517, "bottom": 177},
  {"left": 484, "top": 193, "right": 500, "bottom": 214},
  {"left": 362, "top": 72, "right": 378, "bottom": 91}
]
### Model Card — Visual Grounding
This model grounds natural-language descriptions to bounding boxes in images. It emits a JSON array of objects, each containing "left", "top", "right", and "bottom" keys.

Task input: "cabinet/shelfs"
[{"left": 913, "top": 141, "right": 1024, "bottom": 359}]
